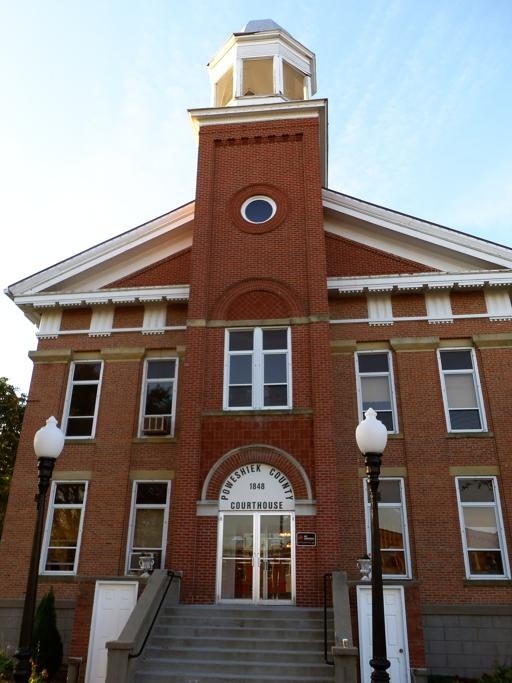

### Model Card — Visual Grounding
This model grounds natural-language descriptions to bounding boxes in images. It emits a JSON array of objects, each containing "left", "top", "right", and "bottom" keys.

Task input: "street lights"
[
  {"left": 355, "top": 405, "right": 394, "bottom": 683},
  {"left": 14, "top": 415, "right": 67, "bottom": 683}
]
